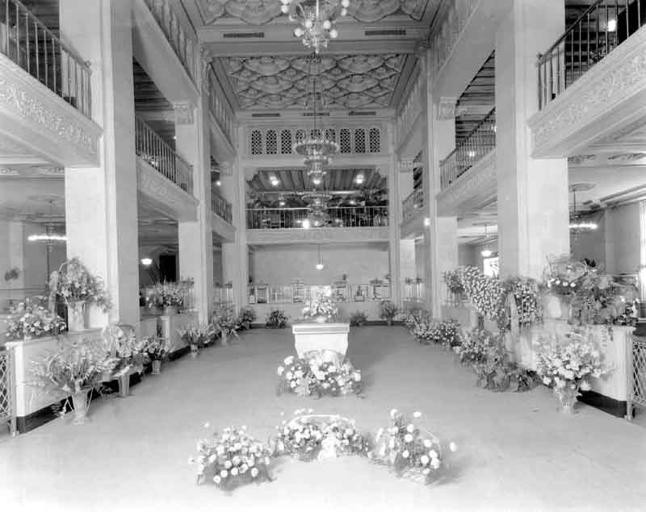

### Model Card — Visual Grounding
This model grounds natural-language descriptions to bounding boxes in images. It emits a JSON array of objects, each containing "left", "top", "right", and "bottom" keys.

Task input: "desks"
[{"left": 9, "top": 328, "right": 104, "bottom": 433}]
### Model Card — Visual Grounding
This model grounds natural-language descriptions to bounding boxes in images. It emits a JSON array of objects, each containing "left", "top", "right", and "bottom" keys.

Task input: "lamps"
[
  {"left": 569, "top": 189, "right": 599, "bottom": 233},
  {"left": 280, "top": 0, "right": 350, "bottom": 229}
]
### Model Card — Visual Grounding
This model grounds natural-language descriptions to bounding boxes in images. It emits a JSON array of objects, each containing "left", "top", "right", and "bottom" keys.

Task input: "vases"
[
  {"left": 387, "top": 317, "right": 392, "bottom": 326},
  {"left": 71, "top": 386, "right": 91, "bottom": 426},
  {"left": 22, "top": 332, "right": 32, "bottom": 340},
  {"left": 69, "top": 298, "right": 86, "bottom": 331},
  {"left": 118, "top": 372, "right": 131, "bottom": 397}
]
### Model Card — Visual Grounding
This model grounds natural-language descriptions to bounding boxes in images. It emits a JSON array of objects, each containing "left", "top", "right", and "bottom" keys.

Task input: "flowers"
[
  {"left": 268, "top": 284, "right": 367, "bottom": 397},
  {"left": 380, "top": 301, "right": 399, "bottom": 315},
  {"left": 102, "top": 323, "right": 147, "bottom": 375},
  {"left": 188, "top": 406, "right": 454, "bottom": 490},
  {"left": 6, "top": 298, "right": 66, "bottom": 332},
  {"left": 148, "top": 260, "right": 255, "bottom": 376},
  {"left": 36, "top": 338, "right": 104, "bottom": 391},
  {"left": 403, "top": 260, "right": 637, "bottom": 421},
  {"left": 47, "top": 258, "right": 111, "bottom": 299}
]
